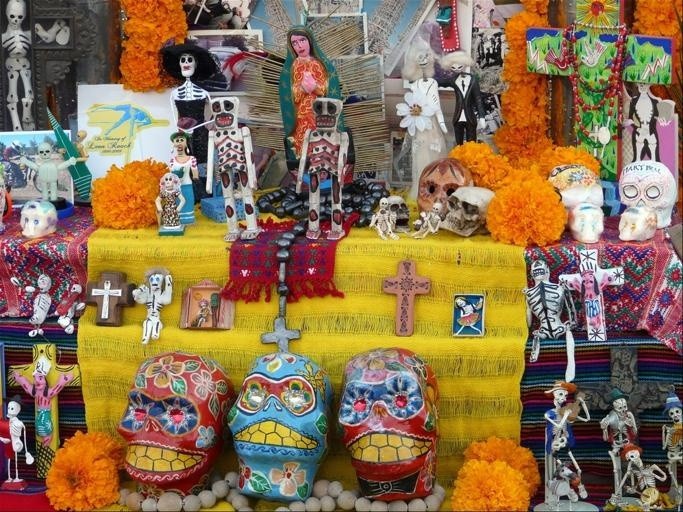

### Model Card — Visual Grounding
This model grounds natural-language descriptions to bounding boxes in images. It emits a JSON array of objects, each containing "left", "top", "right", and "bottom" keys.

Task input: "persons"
[{"left": 473, "top": 29, "right": 505, "bottom": 136}]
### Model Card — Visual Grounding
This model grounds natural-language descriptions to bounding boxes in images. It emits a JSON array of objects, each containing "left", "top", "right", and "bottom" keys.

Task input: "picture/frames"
[
  {"left": 0, "top": 129, "right": 75, "bottom": 208},
  {"left": 187, "top": 29, "right": 264, "bottom": 52},
  {"left": 30, "top": 16, "right": 74, "bottom": 51},
  {"left": 386, "top": 129, "right": 411, "bottom": 188},
  {"left": 245, "top": 0, "right": 437, "bottom": 77},
  {"left": 202, "top": 90, "right": 265, "bottom": 134},
  {"left": 324, "top": 53, "right": 385, "bottom": 121},
  {"left": 299, "top": 11, "right": 368, "bottom": 59}
]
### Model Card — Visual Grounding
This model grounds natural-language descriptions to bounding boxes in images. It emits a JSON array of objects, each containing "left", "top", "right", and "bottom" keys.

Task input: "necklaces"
[{"left": 561, "top": 20, "right": 627, "bottom": 140}]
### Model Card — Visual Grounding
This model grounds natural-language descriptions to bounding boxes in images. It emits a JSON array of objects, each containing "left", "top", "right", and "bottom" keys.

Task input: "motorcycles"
[{"left": 2, "top": 155, "right": 58, "bottom": 193}]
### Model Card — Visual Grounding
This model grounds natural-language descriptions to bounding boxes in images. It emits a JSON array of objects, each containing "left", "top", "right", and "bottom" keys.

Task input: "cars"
[{"left": 198, "top": 45, "right": 247, "bottom": 92}]
